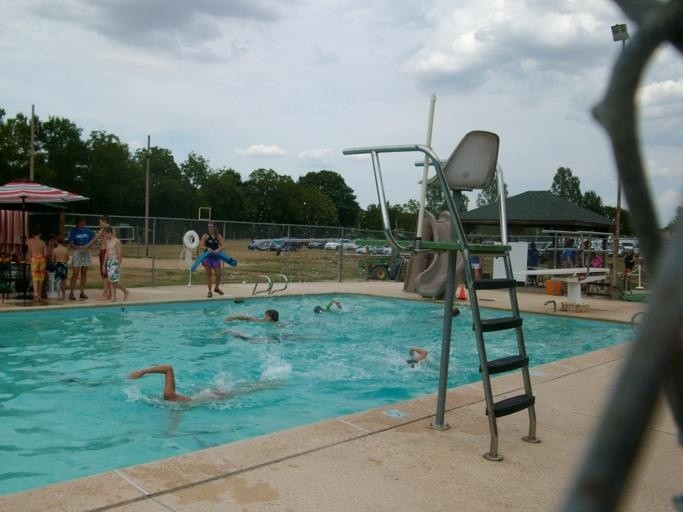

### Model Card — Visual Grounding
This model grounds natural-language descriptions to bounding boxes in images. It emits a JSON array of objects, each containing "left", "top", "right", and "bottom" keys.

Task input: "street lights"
[{"left": 606, "top": 20, "right": 630, "bottom": 283}]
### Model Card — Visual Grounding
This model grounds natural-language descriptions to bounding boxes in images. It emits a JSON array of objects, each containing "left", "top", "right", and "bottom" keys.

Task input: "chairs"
[
  {"left": 0, "top": 255, "right": 50, "bottom": 302},
  {"left": 415, "top": 128, "right": 499, "bottom": 192}
]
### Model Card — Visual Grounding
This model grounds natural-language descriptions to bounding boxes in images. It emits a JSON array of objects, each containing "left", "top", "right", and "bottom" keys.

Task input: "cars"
[
  {"left": 246, "top": 236, "right": 392, "bottom": 255},
  {"left": 589, "top": 236, "right": 642, "bottom": 259}
]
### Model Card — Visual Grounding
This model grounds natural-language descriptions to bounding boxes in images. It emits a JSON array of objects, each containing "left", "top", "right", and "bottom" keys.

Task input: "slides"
[{"left": 415, "top": 208, "right": 466, "bottom": 297}]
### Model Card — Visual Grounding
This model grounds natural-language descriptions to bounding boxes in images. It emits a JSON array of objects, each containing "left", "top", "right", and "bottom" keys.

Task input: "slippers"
[
  {"left": 207, "top": 292, "right": 212, "bottom": 297},
  {"left": 80, "top": 293, "right": 89, "bottom": 299},
  {"left": 69, "top": 294, "right": 76, "bottom": 300},
  {"left": 214, "top": 288, "right": 224, "bottom": 295}
]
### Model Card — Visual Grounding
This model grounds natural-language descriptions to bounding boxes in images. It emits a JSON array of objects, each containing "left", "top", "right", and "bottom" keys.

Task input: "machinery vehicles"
[{"left": 357, "top": 243, "right": 402, "bottom": 281}]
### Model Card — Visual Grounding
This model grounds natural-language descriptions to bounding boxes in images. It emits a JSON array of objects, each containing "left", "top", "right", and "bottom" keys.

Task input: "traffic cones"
[{"left": 458, "top": 285, "right": 466, "bottom": 300}]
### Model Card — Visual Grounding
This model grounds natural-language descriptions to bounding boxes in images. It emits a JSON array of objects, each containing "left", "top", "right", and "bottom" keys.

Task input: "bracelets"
[
  {"left": 205, "top": 247, "right": 209, "bottom": 252},
  {"left": 336, "top": 302, "right": 339, "bottom": 304}
]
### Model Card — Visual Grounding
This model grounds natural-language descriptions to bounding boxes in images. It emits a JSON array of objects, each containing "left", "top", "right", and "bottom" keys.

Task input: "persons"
[
  {"left": 314, "top": 300, "right": 342, "bottom": 314},
  {"left": 407, "top": 347, "right": 427, "bottom": 367},
  {"left": 199, "top": 221, "right": 224, "bottom": 297},
  {"left": 214, "top": 328, "right": 292, "bottom": 343},
  {"left": 165, "top": 410, "right": 180, "bottom": 435},
  {"left": 127, "top": 365, "right": 281, "bottom": 401},
  {"left": 453, "top": 308, "right": 459, "bottom": 316},
  {"left": 26, "top": 215, "right": 130, "bottom": 304},
  {"left": 469, "top": 238, "right": 485, "bottom": 281},
  {"left": 528, "top": 237, "right": 639, "bottom": 291},
  {"left": 224, "top": 309, "right": 285, "bottom": 322}
]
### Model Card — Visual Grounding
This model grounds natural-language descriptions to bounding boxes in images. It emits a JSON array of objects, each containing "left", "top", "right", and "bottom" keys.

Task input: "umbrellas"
[{"left": 0, "top": 179, "right": 91, "bottom": 298}]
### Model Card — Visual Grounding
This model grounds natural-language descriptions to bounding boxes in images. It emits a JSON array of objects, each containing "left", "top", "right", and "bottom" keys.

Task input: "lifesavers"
[{"left": 183, "top": 229, "right": 199, "bottom": 250}]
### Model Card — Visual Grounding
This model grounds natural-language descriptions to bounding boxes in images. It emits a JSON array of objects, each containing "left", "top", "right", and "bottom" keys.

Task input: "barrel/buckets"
[
  {"left": 470, "top": 263, "right": 483, "bottom": 281},
  {"left": 545, "top": 280, "right": 562, "bottom": 296}
]
